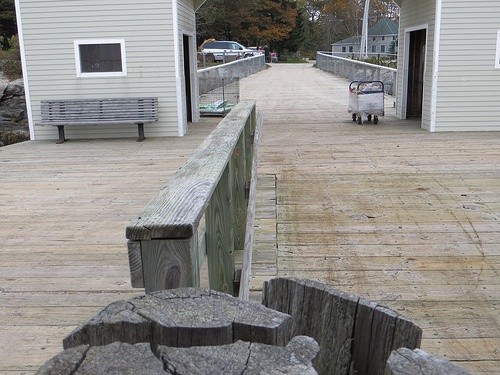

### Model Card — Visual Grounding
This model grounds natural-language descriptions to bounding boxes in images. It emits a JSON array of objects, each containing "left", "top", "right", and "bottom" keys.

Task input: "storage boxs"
[{"left": 198, "top": 76, "right": 240, "bottom": 118}]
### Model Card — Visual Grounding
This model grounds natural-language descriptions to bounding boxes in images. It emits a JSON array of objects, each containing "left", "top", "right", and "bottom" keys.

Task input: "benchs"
[{"left": 35, "top": 97, "right": 159, "bottom": 144}]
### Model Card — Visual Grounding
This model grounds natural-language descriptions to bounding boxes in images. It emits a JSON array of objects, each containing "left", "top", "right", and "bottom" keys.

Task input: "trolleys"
[{"left": 348, "top": 80, "right": 385, "bottom": 125}]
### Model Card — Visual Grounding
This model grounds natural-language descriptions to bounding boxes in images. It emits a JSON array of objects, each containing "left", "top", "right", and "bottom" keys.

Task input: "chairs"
[{"left": 271, "top": 53, "right": 278, "bottom": 63}]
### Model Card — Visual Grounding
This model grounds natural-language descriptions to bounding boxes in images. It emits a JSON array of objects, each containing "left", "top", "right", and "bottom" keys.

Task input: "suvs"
[{"left": 201, "top": 41, "right": 261, "bottom": 63}]
